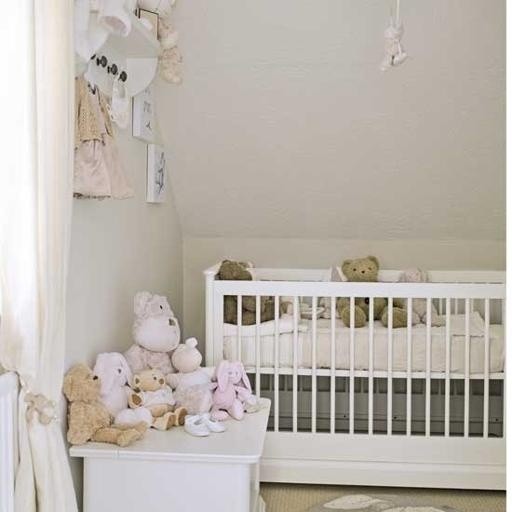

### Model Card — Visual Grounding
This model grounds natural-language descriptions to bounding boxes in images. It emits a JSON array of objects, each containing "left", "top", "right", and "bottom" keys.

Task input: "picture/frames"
[
  {"left": 136, "top": 7, "right": 159, "bottom": 42},
  {"left": 133, "top": 82, "right": 169, "bottom": 203}
]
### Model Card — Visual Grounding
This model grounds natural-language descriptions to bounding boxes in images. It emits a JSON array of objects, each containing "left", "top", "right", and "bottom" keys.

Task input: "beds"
[{"left": 201, "top": 262, "right": 504, "bottom": 491}]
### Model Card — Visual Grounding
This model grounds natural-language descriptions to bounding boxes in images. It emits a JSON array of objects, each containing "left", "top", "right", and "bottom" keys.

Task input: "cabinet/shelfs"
[{"left": 62, "top": 396, "right": 273, "bottom": 512}]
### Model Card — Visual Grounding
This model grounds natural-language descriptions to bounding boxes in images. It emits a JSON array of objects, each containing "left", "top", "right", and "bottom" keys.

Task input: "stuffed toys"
[
  {"left": 219, "top": 260, "right": 284, "bottom": 324},
  {"left": 61, "top": 290, "right": 261, "bottom": 447},
  {"left": 379, "top": 18, "right": 408, "bottom": 73},
  {"left": 335, "top": 255, "right": 408, "bottom": 327},
  {"left": 398, "top": 262, "right": 447, "bottom": 327}
]
